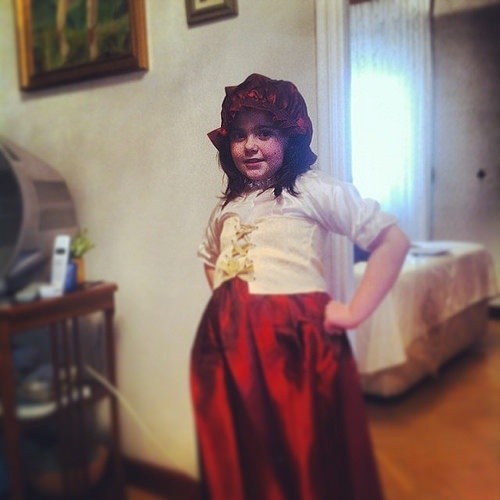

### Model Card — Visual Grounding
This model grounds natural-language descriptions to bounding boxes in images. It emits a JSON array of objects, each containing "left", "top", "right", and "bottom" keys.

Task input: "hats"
[{"left": 204, "top": 67, "right": 313, "bottom": 163}]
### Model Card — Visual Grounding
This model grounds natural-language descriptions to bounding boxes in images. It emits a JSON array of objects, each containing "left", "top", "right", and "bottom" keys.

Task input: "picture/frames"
[
  {"left": 14, "top": 0, "right": 149, "bottom": 92},
  {"left": 184, "top": 0, "right": 237, "bottom": 26}
]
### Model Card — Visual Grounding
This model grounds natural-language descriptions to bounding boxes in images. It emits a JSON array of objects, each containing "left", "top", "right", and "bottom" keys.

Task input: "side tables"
[{"left": 0, "top": 280, "right": 126, "bottom": 500}]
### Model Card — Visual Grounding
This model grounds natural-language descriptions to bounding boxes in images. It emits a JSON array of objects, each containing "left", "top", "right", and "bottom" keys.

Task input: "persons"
[{"left": 187, "top": 73, "right": 410, "bottom": 499}]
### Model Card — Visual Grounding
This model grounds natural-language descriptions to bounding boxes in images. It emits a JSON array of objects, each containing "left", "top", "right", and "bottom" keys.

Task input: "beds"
[{"left": 352, "top": 241, "right": 500, "bottom": 398}]
